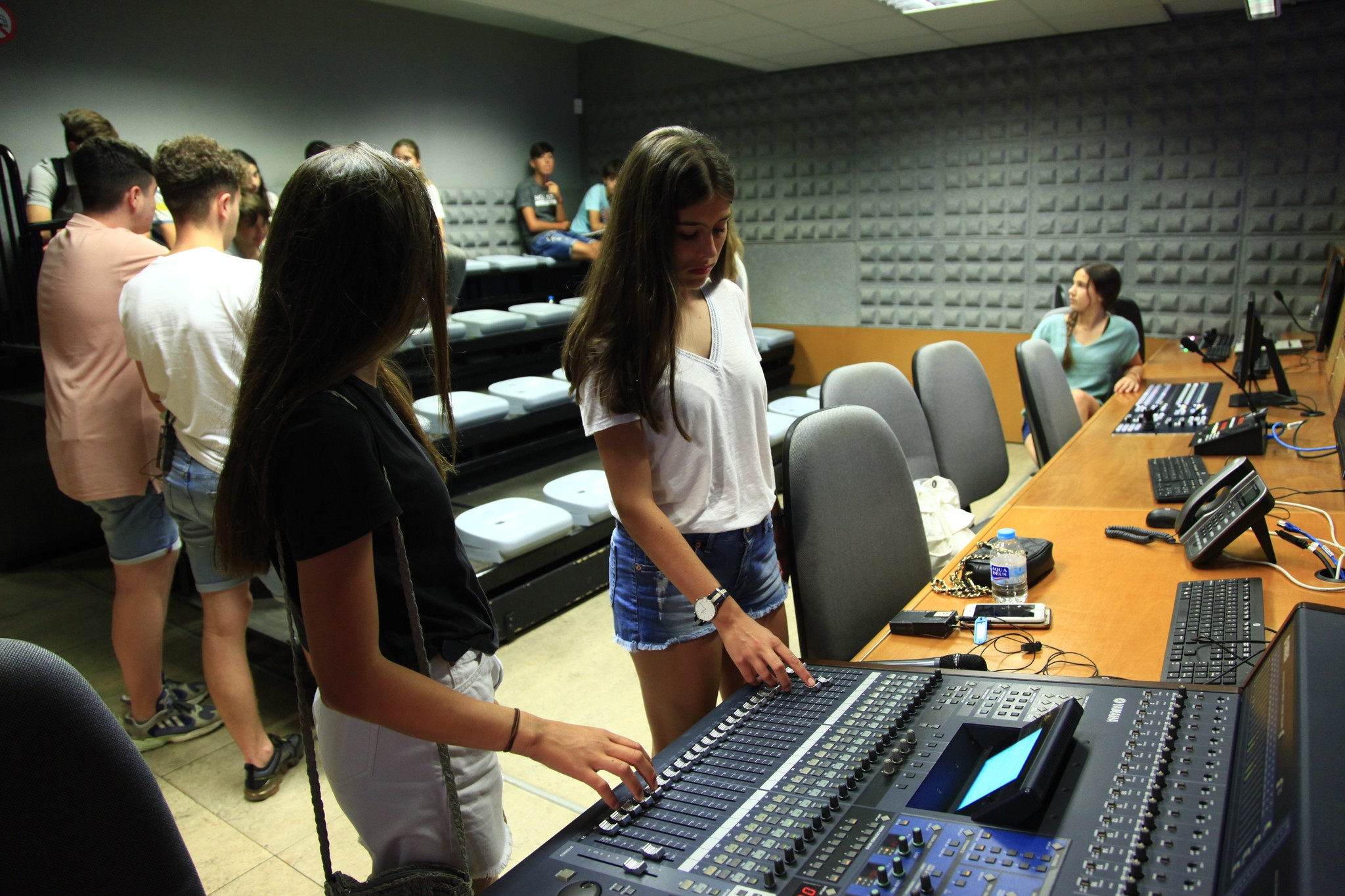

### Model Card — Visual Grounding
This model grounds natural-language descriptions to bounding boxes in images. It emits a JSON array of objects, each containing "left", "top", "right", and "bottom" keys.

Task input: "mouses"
[{"left": 1146, "top": 507, "right": 1180, "bottom": 528}]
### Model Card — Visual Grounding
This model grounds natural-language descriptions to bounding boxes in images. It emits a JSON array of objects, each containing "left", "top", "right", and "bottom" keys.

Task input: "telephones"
[{"left": 1174, "top": 456, "right": 1274, "bottom": 569}]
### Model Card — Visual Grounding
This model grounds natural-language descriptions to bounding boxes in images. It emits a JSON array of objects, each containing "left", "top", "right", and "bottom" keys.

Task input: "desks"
[{"left": 850, "top": 340, "right": 1344, "bottom": 682}]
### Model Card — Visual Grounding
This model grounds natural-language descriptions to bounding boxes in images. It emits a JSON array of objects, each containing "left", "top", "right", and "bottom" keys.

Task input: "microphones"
[
  {"left": 1179, "top": 336, "right": 1256, "bottom": 412},
  {"left": 862, "top": 653, "right": 988, "bottom": 671}
]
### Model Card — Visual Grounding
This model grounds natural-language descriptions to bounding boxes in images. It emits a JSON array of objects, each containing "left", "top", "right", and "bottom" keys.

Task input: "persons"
[
  {"left": 513, "top": 142, "right": 603, "bottom": 264},
  {"left": 561, "top": 126, "right": 817, "bottom": 750},
  {"left": 37, "top": 133, "right": 224, "bottom": 754},
  {"left": 24, "top": 109, "right": 332, "bottom": 260},
  {"left": 570, "top": 159, "right": 626, "bottom": 240},
  {"left": 1020, "top": 263, "right": 1144, "bottom": 465},
  {"left": 120, "top": 136, "right": 304, "bottom": 800},
  {"left": 390, "top": 140, "right": 466, "bottom": 316},
  {"left": 213, "top": 138, "right": 658, "bottom": 891}
]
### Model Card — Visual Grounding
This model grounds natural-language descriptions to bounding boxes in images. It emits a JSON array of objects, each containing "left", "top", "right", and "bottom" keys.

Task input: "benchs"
[{"left": 168, "top": 252, "right": 825, "bottom": 651}]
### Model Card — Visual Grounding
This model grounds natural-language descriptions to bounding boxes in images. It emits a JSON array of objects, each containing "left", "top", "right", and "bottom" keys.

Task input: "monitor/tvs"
[{"left": 1227, "top": 292, "right": 1300, "bottom": 408}]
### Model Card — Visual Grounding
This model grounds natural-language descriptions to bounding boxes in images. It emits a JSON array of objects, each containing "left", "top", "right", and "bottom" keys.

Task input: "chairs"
[
  {"left": 782, "top": 405, "right": 932, "bottom": 661},
  {"left": 1015, "top": 340, "right": 1080, "bottom": 470},
  {"left": 912, "top": 342, "right": 1010, "bottom": 534},
  {"left": 1109, "top": 297, "right": 1145, "bottom": 365},
  {"left": 813, "top": 361, "right": 941, "bottom": 485},
  {"left": 0, "top": 636, "right": 207, "bottom": 895}
]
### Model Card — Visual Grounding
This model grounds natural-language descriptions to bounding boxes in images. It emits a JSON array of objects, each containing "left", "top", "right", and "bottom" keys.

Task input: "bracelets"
[
  {"left": 558, "top": 199, "right": 563, "bottom": 204},
  {"left": 1124, "top": 371, "right": 1141, "bottom": 384},
  {"left": 503, "top": 708, "right": 520, "bottom": 752}
]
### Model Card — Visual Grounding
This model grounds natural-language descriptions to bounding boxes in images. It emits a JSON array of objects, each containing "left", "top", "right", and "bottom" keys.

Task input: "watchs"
[{"left": 694, "top": 586, "right": 731, "bottom": 626}]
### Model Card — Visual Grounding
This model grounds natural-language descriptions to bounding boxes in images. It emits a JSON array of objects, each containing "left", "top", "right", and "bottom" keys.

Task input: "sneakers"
[
  {"left": 119, "top": 669, "right": 225, "bottom": 753},
  {"left": 243, "top": 733, "right": 305, "bottom": 803}
]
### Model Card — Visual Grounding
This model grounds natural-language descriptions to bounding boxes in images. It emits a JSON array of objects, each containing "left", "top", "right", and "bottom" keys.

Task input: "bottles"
[{"left": 989, "top": 528, "right": 1028, "bottom": 605}]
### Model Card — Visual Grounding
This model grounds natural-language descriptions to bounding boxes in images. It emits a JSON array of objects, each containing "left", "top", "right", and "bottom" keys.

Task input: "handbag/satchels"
[{"left": 324, "top": 862, "right": 474, "bottom": 896}]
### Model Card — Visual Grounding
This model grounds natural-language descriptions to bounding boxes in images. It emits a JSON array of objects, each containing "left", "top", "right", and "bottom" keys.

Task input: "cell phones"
[{"left": 960, "top": 603, "right": 1050, "bottom": 629}]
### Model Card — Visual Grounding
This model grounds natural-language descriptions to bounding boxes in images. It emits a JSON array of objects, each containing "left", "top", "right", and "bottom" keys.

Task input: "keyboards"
[
  {"left": 1148, "top": 453, "right": 1217, "bottom": 502},
  {"left": 1160, "top": 576, "right": 1263, "bottom": 687},
  {"left": 1202, "top": 333, "right": 1236, "bottom": 363}
]
